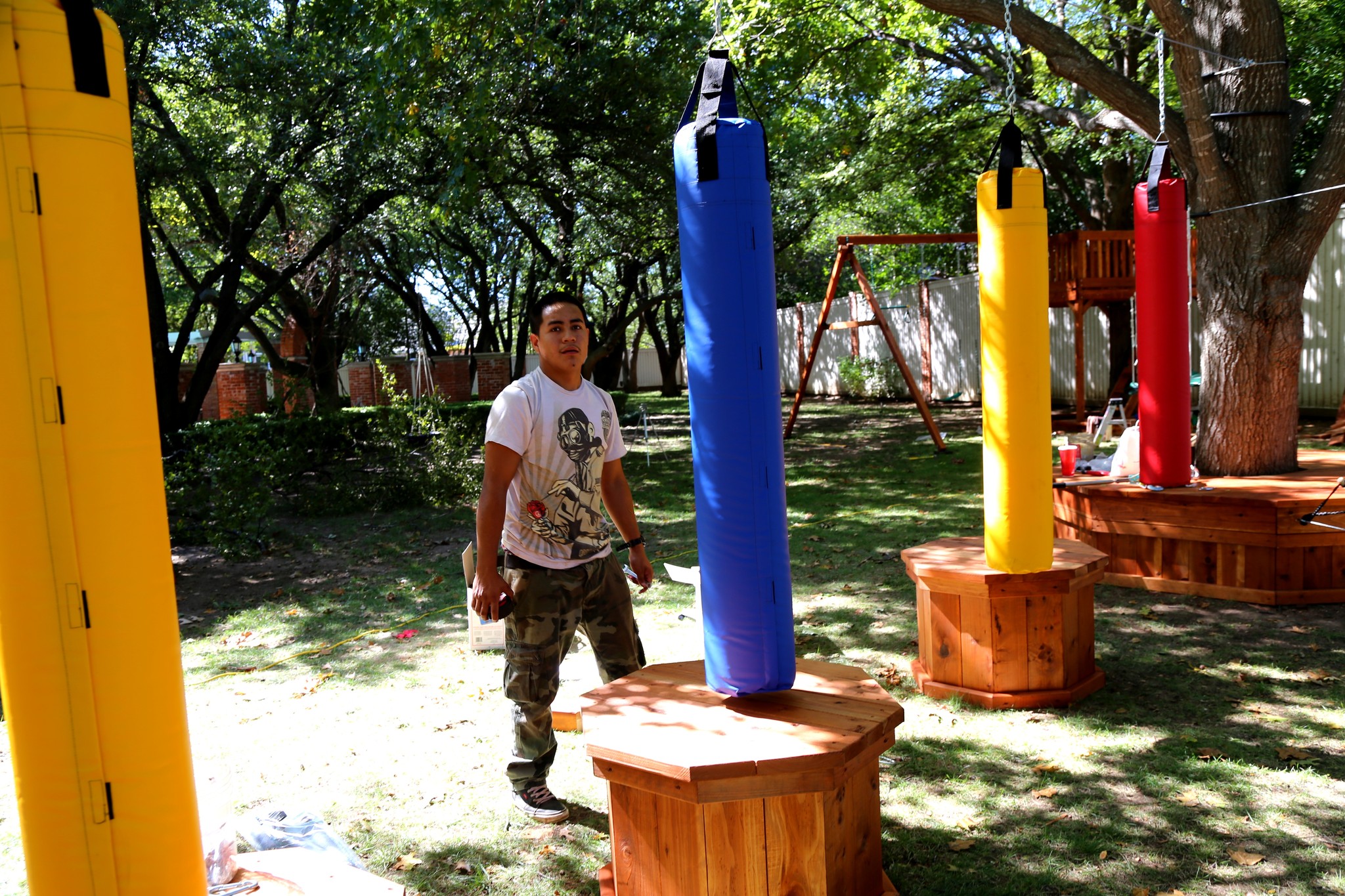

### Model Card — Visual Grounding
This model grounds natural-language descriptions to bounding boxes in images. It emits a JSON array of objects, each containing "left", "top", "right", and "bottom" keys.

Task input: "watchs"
[{"left": 615, "top": 534, "right": 646, "bottom": 553}]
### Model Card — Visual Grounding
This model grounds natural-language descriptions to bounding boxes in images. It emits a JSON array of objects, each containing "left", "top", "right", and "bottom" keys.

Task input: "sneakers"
[{"left": 512, "top": 784, "right": 569, "bottom": 823}]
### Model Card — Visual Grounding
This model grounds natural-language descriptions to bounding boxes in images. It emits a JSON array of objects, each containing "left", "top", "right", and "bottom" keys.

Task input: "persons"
[{"left": 473, "top": 292, "right": 655, "bottom": 822}]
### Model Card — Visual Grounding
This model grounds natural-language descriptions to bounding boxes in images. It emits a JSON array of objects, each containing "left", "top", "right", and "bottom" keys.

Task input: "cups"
[{"left": 1058, "top": 445, "right": 1078, "bottom": 477}]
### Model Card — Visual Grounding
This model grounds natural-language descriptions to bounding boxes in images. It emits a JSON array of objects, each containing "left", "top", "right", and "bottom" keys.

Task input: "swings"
[{"left": 405, "top": 343, "right": 448, "bottom": 446}]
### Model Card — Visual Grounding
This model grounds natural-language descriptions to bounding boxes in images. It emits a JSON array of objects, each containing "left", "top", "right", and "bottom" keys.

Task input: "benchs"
[{"left": 1053, "top": 451, "right": 1345, "bottom": 605}]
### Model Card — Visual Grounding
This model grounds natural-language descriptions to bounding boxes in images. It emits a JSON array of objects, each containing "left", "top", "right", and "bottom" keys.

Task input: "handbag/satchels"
[{"left": 1110, "top": 419, "right": 1140, "bottom": 477}]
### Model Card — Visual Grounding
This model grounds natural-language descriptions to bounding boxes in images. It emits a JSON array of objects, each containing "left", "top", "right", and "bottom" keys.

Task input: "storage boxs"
[{"left": 462, "top": 541, "right": 506, "bottom": 651}]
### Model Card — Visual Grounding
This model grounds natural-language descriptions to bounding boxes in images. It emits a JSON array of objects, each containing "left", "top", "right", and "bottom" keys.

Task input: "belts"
[{"left": 505, "top": 555, "right": 548, "bottom": 571}]
